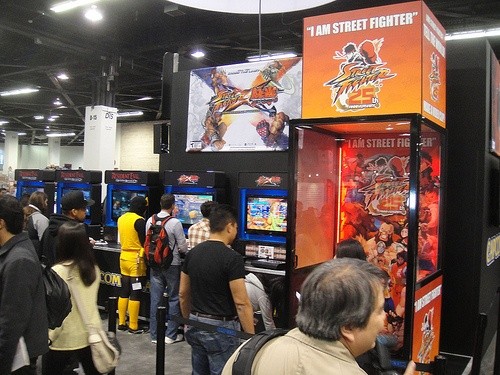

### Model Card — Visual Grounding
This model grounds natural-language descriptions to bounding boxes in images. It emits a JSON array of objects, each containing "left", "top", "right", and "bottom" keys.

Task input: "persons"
[
  {"left": 0, "top": 196, "right": 48, "bottom": 375},
  {"left": 384, "top": 297, "right": 395, "bottom": 315},
  {"left": 187, "top": 201, "right": 221, "bottom": 255},
  {"left": 0, "top": 188, "right": 7, "bottom": 196},
  {"left": 41, "top": 221, "right": 104, "bottom": 375},
  {"left": 118, "top": 196, "right": 148, "bottom": 335},
  {"left": 243, "top": 272, "right": 286, "bottom": 335},
  {"left": 220, "top": 258, "right": 421, "bottom": 375},
  {"left": 37, "top": 190, "right": 96, "bottom": 268},
  {"left": 23, "top": 191, "right": 50, "bottom": 242},
  {"left": 145, "top": 194, "right": 187, "bottom": 344},
  {"left": 333, "top": 238, "right": 366, "bottom": 263},
  {"left": 179, "top": 205, "right": 254, "bottom": 375},
  {"left": 390, "top": 252, "right": 407, "bottom": 308}
]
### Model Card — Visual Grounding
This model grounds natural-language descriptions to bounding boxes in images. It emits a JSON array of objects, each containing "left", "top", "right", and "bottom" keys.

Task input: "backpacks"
[{"left": 144, "top": 214, "right": 177, "bottom": 270}]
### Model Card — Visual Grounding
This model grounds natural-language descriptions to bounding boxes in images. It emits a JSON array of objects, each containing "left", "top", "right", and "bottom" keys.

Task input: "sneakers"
[
  {"left": 118, "top": 323, "right": 129, "bottom": 332},
  {"left": 151, "top": 340, "right": 157, "bottom": 343},
  {"left": 128, "top": 327, "right": 148, "bottom": 334},
  {"left": 165, "top": 334, "right": 183, "bottom": 344}
]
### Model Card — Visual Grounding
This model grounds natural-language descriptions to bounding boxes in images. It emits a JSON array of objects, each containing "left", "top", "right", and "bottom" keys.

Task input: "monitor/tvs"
[
  {"left": 104, "top": 184, "right": 161, "bottom": 228},
  {"left": 57, "top": 183, "right": 102, "bottom": 225},
  {"left": 16, "top": 180, "right": 54, "bottom": 215},
  {"left": 164, "top": 185, "right": 226, "bottom": 237},
  {"left": 239, "top": 188, "right": 288, "bottom": 243}
]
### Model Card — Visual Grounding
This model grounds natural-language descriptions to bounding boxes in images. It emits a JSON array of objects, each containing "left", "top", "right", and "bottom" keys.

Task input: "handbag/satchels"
[
  {"left": 41, "top": 262, "right": 73, "bottom": 330},
  {"left": 88, "top": 330, "right": 122, "bottom": 373}
]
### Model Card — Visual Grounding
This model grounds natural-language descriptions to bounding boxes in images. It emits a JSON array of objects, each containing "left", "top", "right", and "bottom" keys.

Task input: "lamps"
[
  {"left": 246, "top": 50, "right": 298, "bottom": 62},
  {"left": 445, "top": 25, "right": 500, "bottom": 41}
]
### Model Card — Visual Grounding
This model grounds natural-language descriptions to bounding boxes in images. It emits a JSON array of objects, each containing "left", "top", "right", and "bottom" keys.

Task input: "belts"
[{"left": 191, "top": 311, "right": 239, "bottom": 321}]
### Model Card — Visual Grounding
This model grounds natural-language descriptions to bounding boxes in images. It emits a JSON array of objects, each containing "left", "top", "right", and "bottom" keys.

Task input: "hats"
[{"left": 62, "top": 190, "right": 95, "bottom": 210}]
